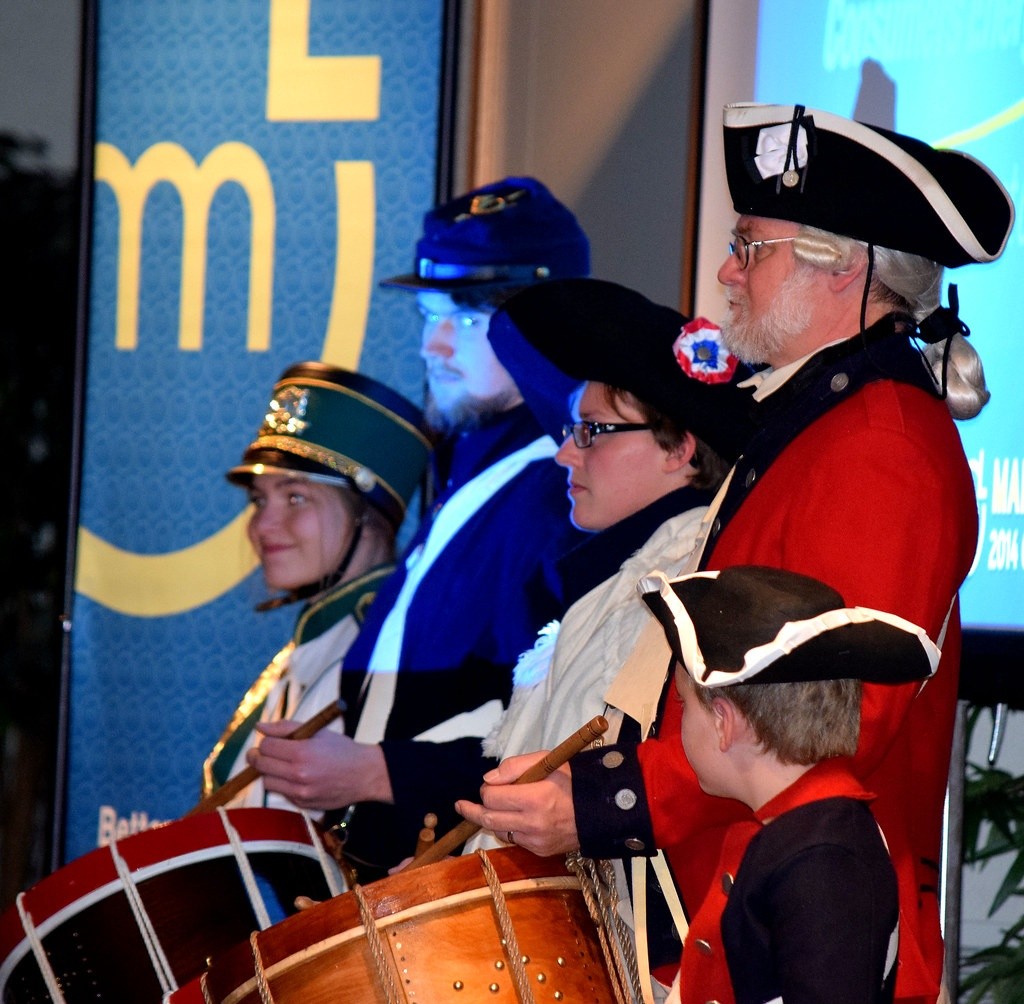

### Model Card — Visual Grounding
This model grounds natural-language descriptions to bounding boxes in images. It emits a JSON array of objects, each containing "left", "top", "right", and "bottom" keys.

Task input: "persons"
[
  {"left": 636, "top": 564, "right": 941, "bottom": 1004},
  {"left": 203, "top": 102, "right": 1016, "bottom": 1004}
]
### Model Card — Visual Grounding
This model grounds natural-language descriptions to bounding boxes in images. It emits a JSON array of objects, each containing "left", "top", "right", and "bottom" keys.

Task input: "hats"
[
  {"left": 380, "top": 176, "right": 591, "bottom": 294},
  {"left": 502, "top": 277, "right": 771, "bottom": 466},
  {"left": 723, "top": 102, "right": 1016, "bottom": 269},
  {"left": 636, "top": 565, "right": 942, "bottom": 689},
  {"left": 225, "top": 361, "right": 436, "bottom": 539}
]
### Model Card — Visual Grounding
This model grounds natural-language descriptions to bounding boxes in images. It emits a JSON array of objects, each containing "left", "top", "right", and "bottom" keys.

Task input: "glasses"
[
  {"left": 562, "top": 421, "right": 652, "bottom": 448},
  {"left": 729, "top": 235, "right": 795, "bottom": 271}
]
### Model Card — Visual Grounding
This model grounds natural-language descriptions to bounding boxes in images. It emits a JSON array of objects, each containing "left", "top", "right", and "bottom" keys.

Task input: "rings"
[{"left": 508, "top": 832, "right": 515, "bottom": 844}]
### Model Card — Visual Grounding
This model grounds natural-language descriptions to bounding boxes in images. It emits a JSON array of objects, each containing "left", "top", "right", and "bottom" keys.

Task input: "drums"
[
  {"left": 203, "top": 844, "right": 638, "bottom": 1004},
  {"left": 0, "top": 806, "right": 361, "bottom": 1003}
]
